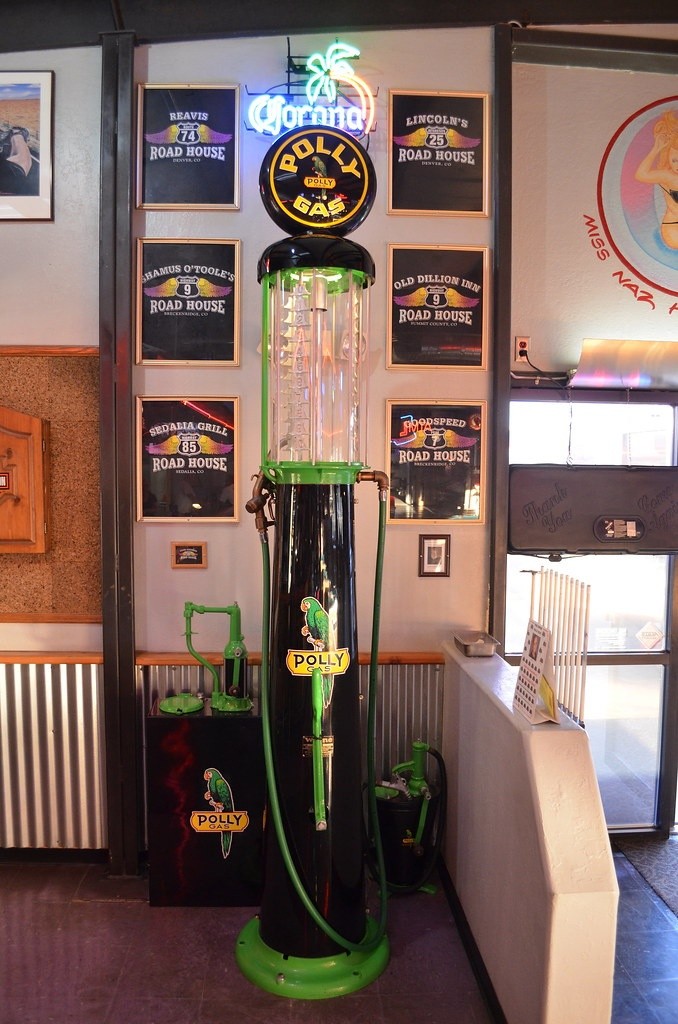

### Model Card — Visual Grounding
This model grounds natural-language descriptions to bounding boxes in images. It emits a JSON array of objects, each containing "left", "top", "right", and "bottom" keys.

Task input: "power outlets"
[{"left": 514, "top": 335, "right": 531, "bottom": 363}]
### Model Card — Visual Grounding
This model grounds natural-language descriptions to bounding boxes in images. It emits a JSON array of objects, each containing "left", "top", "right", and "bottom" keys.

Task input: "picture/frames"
[
  {"left": 133, "top": 82, "right": 241, "bottom": 210},
  {"left": 417, "top": 533, "right": 451, "bottom": 578},
  {"left": 171, "top": 539, "right": 208, "bottom": 569},
  {"left": 383, "top": 398, "right": 489, "bottom": 526},
  {"left": 384, "top": 242, "right": 492, "bottom": 374},
  {"left": 0, "top": 69, "right": 55, "bottom": 224},
  {"left": 135, "top": 394, "right": 241, "bottom": 523},
  {"left": 385, "top": 88, "right": 492, "bottom": 218},
  {"left": 134, "top": 236, "right": 241, "bottom": 367}
]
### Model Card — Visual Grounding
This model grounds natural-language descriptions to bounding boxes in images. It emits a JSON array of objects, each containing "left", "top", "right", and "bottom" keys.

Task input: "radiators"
[{"left": 1, "top": 647, "right": 446, "bottom": 865}]
